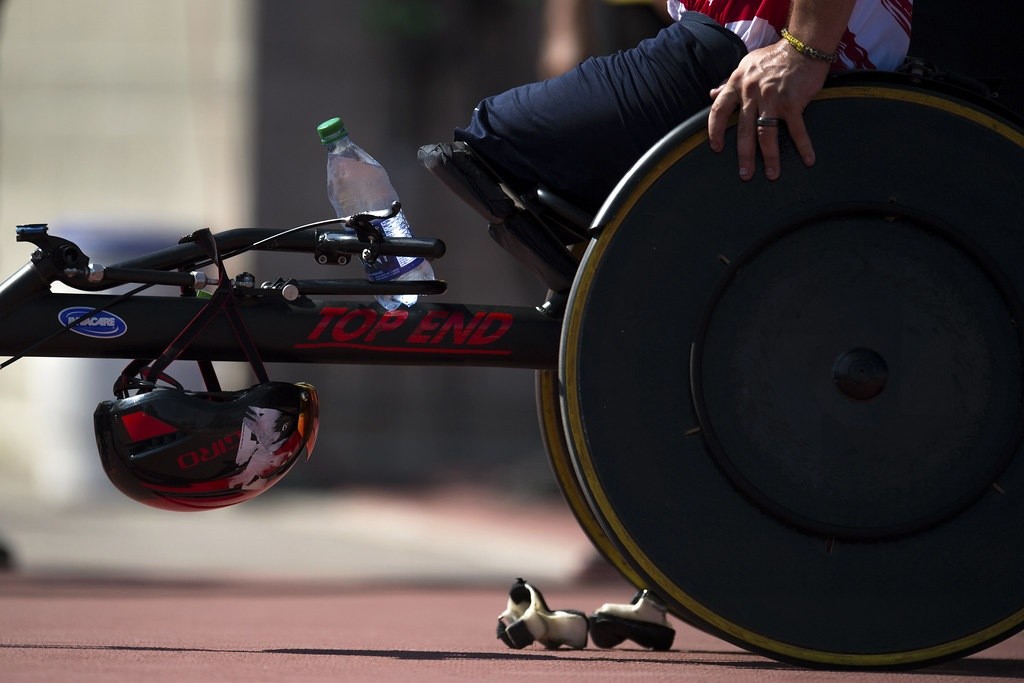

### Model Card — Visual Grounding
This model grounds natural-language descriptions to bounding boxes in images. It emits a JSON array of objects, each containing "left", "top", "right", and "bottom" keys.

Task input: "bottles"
[{"left": 317, "top": 117, "right": 435, "bottom": 311}]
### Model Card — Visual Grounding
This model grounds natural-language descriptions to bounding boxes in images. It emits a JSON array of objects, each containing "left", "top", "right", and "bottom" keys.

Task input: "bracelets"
[{"left": 780, "top": 28, "right": 837, "bottom": 64}]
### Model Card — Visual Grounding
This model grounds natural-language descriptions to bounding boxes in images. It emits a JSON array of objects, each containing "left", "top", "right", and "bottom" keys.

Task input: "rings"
[{"left": 758, "top": 118, "right": 779, "bottom": 127}]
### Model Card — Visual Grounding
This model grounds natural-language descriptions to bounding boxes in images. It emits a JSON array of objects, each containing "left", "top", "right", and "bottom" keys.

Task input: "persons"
[{"left": 453, "top": 0, "right": 913, "bottom": 246}]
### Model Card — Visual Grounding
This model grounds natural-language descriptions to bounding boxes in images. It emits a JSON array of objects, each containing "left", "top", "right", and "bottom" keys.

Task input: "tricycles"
[{"left": 0, "top": 75, "right": 1023, "bottom": 671}]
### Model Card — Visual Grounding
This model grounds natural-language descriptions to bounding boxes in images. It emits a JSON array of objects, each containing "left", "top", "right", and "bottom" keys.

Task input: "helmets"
[{"left": 94, "top": 381, "right": 322, "bottom": 513}]
